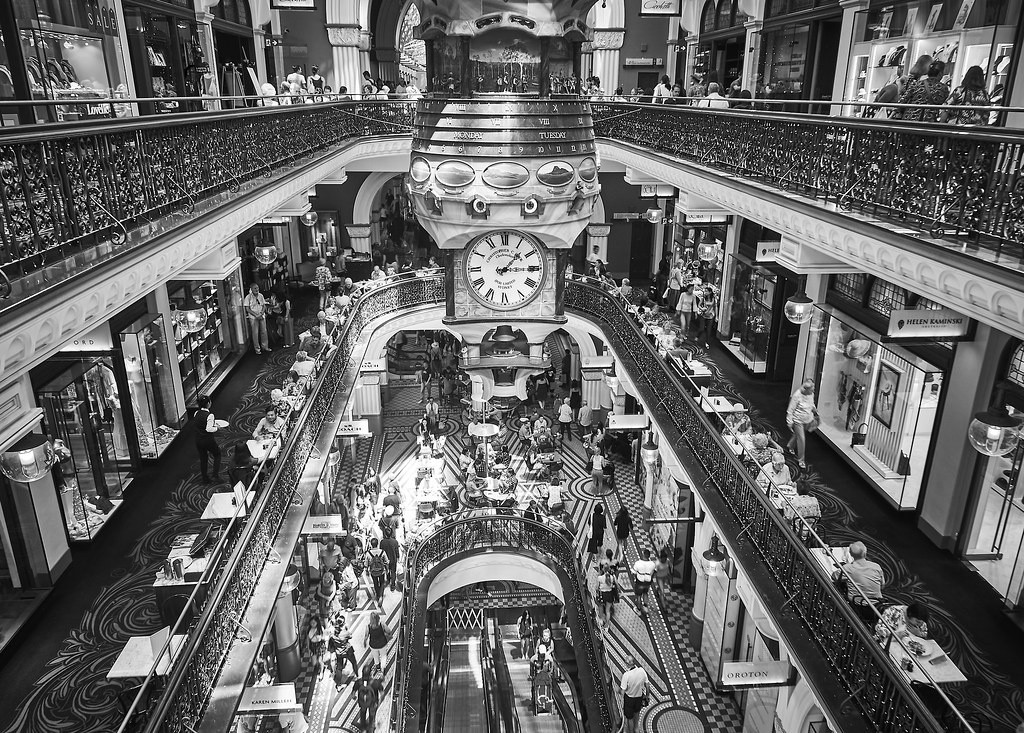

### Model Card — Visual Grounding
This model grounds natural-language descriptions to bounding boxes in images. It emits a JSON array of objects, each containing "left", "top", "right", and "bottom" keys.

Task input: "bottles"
[
  {"left": 163, "top": 558, "right": 172, "bottom": 580},
  {"left": 173, "top": 558, "right": 184, "bottom": 581}
]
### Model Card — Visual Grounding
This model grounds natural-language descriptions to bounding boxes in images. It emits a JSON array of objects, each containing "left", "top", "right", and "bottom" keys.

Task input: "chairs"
[
  {"left": 227, "top": 466, "right": 257, "bottom": 490},
  {"left": 872, "top": 603, "right": 901, "bottom": 633},
  {"left": 551, "top": 461, "right": 564, "bottom": 477},
  {"left": 802, "top": 524, "right": 826, "bottom": 588},
  {"left": 523, "top": 452, "right": 540, "bottom": 483},
  {"left": 910, "top": 680, "right": 955, "bottom": 724},
  {"left": 852, "top": 596, "right": 890, "bottom": 642},
  {"left": 787, "top": 516, "right": 821, "bottom": 569},
  {"left": 418, "top": 504, "right": 435, "bottom": 522}
]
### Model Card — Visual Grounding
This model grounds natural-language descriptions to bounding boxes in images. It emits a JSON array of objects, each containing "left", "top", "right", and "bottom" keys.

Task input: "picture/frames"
[{"left": 871, "top": 359, "right": 907, "bottom": 433}]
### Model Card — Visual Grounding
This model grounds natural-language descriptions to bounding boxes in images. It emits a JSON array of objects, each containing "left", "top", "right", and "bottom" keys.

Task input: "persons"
[
  {"left": 103, "top": 356, "right": 150, "bottom": 456},
  {"left": 578, "top": 54, "right": 989, "bottom": 159},
  {"left": 260, "top": 65, "right": 408, "bottom": 104},
  {"left": 192, "top": 190, "right": 928, "bottom": 733},
  {"left": 431, "top": 71, "right": 577, "bottom": 95},
  {"left": 879, "top": 41, "right": 958, "bottom": 87},
  {"left": 981, "top": 55, "right": 1010, "bottom": 103},
  {"left": 52, "top": 439, "right": 82, "bottom": 531},
  {"left": 0, "top": 56, "right": 78, "bottom": 86}
]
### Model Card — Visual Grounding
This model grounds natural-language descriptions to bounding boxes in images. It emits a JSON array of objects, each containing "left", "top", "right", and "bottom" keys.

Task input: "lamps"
[
  {"left": 328, "top": 446, "right": 340, "bottom": 466},
  {"left": 640, "top": 425, "right": 659, "bottom": 462},
  {"left": 646, "top": 185, "right": 662, "bottom": 224},
  {"left": 606, "top": 360, "right": 619, "bottom": 388},
  {"left": 967, "top": 380, "right": 1019, "bottom": 457},
  {"left": 784, "top": 275, "right": 814, "bottom": 324},
  {"left": 254, "top": 219, "right": 277, "bottom": 264},
  {"left": 0, "top": 430, "right": 55, "bottom": 482},
  {"left": 280, "top": 564, "right": 300, "bottom": 592},
  {"left": 300, "top": 196, "right": 318, "bottom": 226},
  {"left": 697, "top": 228, "right": 718, "bottom": 261},
  {"left": 701, "top": 533, "right": 727, "bottom": 576},
  {"left": 175, "top": 280, "right": 207, "bottom": 332}
]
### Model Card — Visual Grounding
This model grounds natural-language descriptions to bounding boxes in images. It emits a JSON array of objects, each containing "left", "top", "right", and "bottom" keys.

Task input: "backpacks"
[
  {"left": 368, "top": 549, "right": 384, "bottom": 577},
  {"left": 358, "top": 683, "right": 375, "bottom": 707}
]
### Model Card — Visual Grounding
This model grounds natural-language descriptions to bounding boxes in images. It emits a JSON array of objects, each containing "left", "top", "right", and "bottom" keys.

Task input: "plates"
[
  {"left": 902, "top": 637, "right": 933, "bottom": 656},
  {"left": 170, "top": 555, "right": 193, "bottom": 569},
  {"left": 214, "top": 419, "right": 230, "bottom": 428}
]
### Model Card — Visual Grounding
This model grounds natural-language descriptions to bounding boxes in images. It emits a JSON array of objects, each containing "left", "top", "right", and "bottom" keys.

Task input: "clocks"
[{"left": 462, "top": 229, "right": 548, "bottom": 311}]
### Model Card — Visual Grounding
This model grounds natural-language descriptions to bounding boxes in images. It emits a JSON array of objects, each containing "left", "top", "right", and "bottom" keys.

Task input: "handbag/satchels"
[
  {"left": 587, "top": 513, "right": 593, "bottom": 539},
  {"left": 381, "top": 624, "right": 393, "bottom": 643},
  {"left": 850, "top": 423, "right": 868, "bottom": 448},
  {"left": 586, "top": 455, "right": 594, "bottom": 471}
]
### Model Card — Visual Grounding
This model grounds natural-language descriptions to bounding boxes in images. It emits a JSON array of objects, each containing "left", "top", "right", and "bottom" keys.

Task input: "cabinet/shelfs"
[
  {"left": 169, "top": 289, "right": 225, "bottom": 399},
  {"left": 694, "top": 46, "right": 712, "bottom": 82}
]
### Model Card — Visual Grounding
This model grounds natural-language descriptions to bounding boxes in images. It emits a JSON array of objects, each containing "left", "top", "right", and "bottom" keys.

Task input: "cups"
[{"left": 823, "top": 544, "right": 828, "bottom": 554}]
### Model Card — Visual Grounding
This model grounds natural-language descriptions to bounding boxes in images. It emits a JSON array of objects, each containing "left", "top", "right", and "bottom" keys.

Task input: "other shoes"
[
  {"left": 790, "top": 449, "right": 795, "bottom": 454},
  {"left": 634, "top": 608, "right": 642, "bottom": 615},
  {"left": 799, "top": 462, "right": 806, "bottom": 468},
  {"left": 319, "top": 672, "right": 323, "bottom": 679},
  {"left": 337, "top": 685, "right": 345, "bottom": 691},
  {"left": 347, "top": 607, "right": 355, "bottom": 612},
  {"left": 643, "top": 605, "right": 649, "bottom": 613}
]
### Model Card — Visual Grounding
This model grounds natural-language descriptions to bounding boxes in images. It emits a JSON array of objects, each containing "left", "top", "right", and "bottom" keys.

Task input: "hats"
[
  {"left": 730, "top": 403, "right": 748, "bottom": 413},
  {"left": 539, "top": 645, "right": 547, "bottom": 654}
]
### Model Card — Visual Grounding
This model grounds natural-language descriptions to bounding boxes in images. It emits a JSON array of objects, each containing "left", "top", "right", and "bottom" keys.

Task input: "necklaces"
[
  {"left": 878, "top": 45, "right": 958, "bottom": 87},
  {"left": 887, "top": 49, "right": 896, "bottom": 58},
  {"left": 879, "top": 58, "right": 885, "bottom": 64},
  {"left": 989, "top": 87, "right": 1003, "bottom": 97},
  {"left": 892, "top": 48, "right": 904, "bottom": 62}
]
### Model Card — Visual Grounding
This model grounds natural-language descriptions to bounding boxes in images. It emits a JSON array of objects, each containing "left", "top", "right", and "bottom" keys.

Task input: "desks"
[
  {"left": 106, "top": 634, "right": 189, "bottom": 721},
  {"left": 809, "top": 547, "right": 854, "bottom": 628},
  {"left": 878, "top": 628, "right": 968, "bottom": 685},
  {"left": 628, "top": 304, "right": 800, "bottom": 510},
  {"left": 153, "top": 531, "right": 226, "bottom": 636},
  {"left": 289, "top": 361, "right": 323, "bottom": 379},
  {"left": 285, "top": 395, "right": 306, "bottom": 411},
  {"left": 347, "top": 258, "right": 372, "bottom": 278},
  {"left": 537, "top": 452, "right": 560, "bottom": 477},
  {"left": 246, "top": 437, "right": 281, "bottom": 471},
  {"left": 200, "top": 491, "right": 256, "bottom": 532}
]
